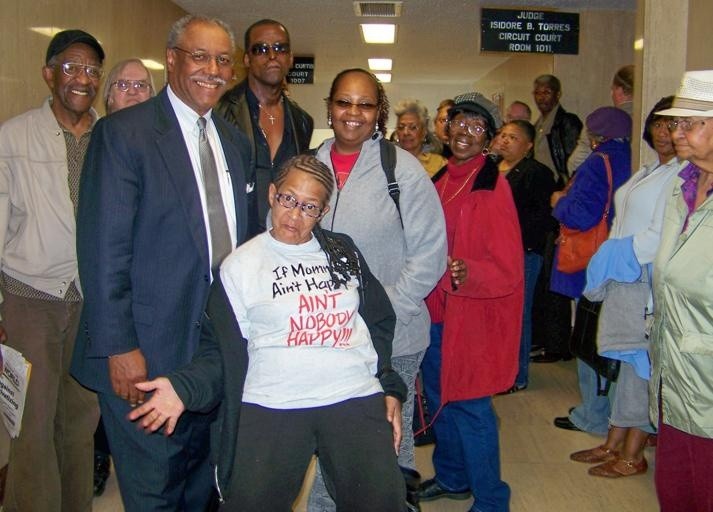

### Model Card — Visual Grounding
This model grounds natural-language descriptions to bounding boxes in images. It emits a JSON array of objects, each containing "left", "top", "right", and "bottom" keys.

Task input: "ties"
[{"left": 197, "top": 116, "right": 232, "bottom": 279}]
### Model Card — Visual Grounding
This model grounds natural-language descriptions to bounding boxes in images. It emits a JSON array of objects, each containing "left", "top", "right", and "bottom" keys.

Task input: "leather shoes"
[
  {"left": 570, "top": 445, "right": 618, "bottom": 463},
  {"left": 554, "top": 417, "right": 579, "bottom": 430},
  {"left": 93, "top": 458, "right": 111, "bottom": 495},
  {"left": 418, "top": 478, "right": 471, "bottom": 501},
  {"left": 589, "top": 458, "right": 647, "bottom": 477}
]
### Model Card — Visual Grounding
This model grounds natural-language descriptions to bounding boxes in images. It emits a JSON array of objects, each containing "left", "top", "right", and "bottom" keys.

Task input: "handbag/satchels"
[
  {"left": 568, "top": 296, "right": 621, "bottom": 395},
  {"left": 555, "top": 216, "right": 613, "bottom": 273}
]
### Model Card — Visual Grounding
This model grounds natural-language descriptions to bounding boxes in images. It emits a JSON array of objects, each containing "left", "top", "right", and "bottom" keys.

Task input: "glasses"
[
  {"left": 110, "top": 80, "right": 151, "bottom": 91},
  {"left": 668, "top": 120, "right": 705, "bottom": 130},
  {"left": 49, "top": 62, "right": 104, "bottom": 78},
  {"left": 532, "top": 89, "right": 551, "bottom": 95},
  {"left": 449, "top": 120, "right": 486, "bottom": 137},
  {"left": 251, "top": 42, "right": 290, "bottom": 55},
  {"left": 333, "top": 99, "right": 379, "bottom": 109},
  {"left": 397, "top": 124, "right": 419, "bottom": 131},
  {"left": 275, "top": 191, "right": 323, "bottom": 218},
  {"left": 175, "top": 46, "right": 233, "bottom": 66}
]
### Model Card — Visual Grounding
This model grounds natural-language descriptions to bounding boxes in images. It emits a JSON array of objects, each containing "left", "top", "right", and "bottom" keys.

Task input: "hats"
[
  {"left": 613, "top": 66, "right": 633, "bottom": 90},
  {"left": 447, "top": 92, "right": 502, "bottom": 139},
  {"left": 653, "top": 70, "right": 713, "bottom": 117},
  {"left": 46, "top": 30, "right": 104, "bottom": 63},
  {"left": 586, "top": 106, "right": 632, "bottom": 139}
]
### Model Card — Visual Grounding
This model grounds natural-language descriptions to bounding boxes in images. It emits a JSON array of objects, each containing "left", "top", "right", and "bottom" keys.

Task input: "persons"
[
  {"left": 552, "top": 64, "right": 684, "bottom": 476},
  {"left": 649, "top": 70, "right": 713, "bottom": 511},
  {"left": 491, "top": 73, "right": 585, "bottom": 396},
  {"left": 1, "top": 29, "right": 155, "bottom": 511},
  {"left": 267, "top": 67, "right": 525, "bottom": 512},
  {"left": 69, "top": 17, "right": 252, "bottom": 511},
  {"left": 128, "top": 153, "right": 407, "bottom": 512},
  {"left": 221, "top": 16, "right": 312, "bottom": 236}
]
